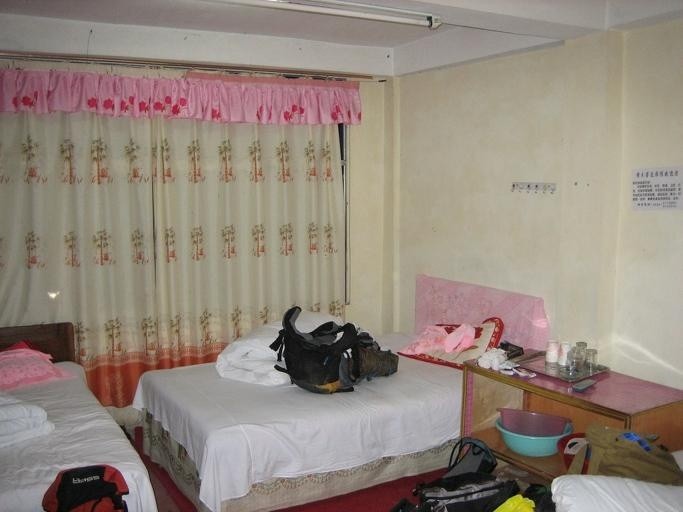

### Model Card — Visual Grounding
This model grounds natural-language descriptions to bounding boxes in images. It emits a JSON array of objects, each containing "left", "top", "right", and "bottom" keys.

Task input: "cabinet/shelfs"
[{"left": 458, "top": 347, "right": 682, "bottom": 498}]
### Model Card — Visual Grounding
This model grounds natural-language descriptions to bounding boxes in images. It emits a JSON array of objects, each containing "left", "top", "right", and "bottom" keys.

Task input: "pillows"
[
  {"left": 396, "top": 316, "right": 504, "bottom": 370},
  {"left": 0, "top": 339, "right": 34, "bottom": 351},
  {"left": 0, "top": 349, "right": 75, "bottom": 390}
]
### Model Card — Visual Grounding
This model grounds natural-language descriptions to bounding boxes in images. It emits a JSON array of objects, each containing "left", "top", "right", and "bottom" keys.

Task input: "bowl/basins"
[{"left": 494, "top": 407, "right": 574, "bottom": 457}]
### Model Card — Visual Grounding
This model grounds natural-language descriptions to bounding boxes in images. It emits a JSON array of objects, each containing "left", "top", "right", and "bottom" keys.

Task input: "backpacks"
[
  {"left": 42, "top": 464, "right": 130, "bottom": 512},
  {"left": 269, "top": 306, "right": 400, "bottom": 396},
  {"left": 388, "top": 436, "right": 556, "bottom": 512},
  {"left": 568, "top": 424, "right": 683, "bottom": 488}
]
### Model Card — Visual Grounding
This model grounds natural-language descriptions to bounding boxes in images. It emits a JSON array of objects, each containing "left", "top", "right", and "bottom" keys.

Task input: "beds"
[
  {"left": 0, "top": 320, "right": 158, "bottom": 511},
  {"left": 543, "top": 450, "right": 682, "bottom": 511},
  {"left": 127, "top": 272, "right": 547, "bottom": 511}
]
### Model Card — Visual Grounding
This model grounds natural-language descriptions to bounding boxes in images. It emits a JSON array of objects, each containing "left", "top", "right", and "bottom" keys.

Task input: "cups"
[{"left": 545, "top": 339, "right": 597, "bottom": 379}]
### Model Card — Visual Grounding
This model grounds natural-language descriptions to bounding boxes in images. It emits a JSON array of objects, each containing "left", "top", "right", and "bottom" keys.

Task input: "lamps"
[{"left": 237, "top": 0, "right": 439, "bottom": 30}]
